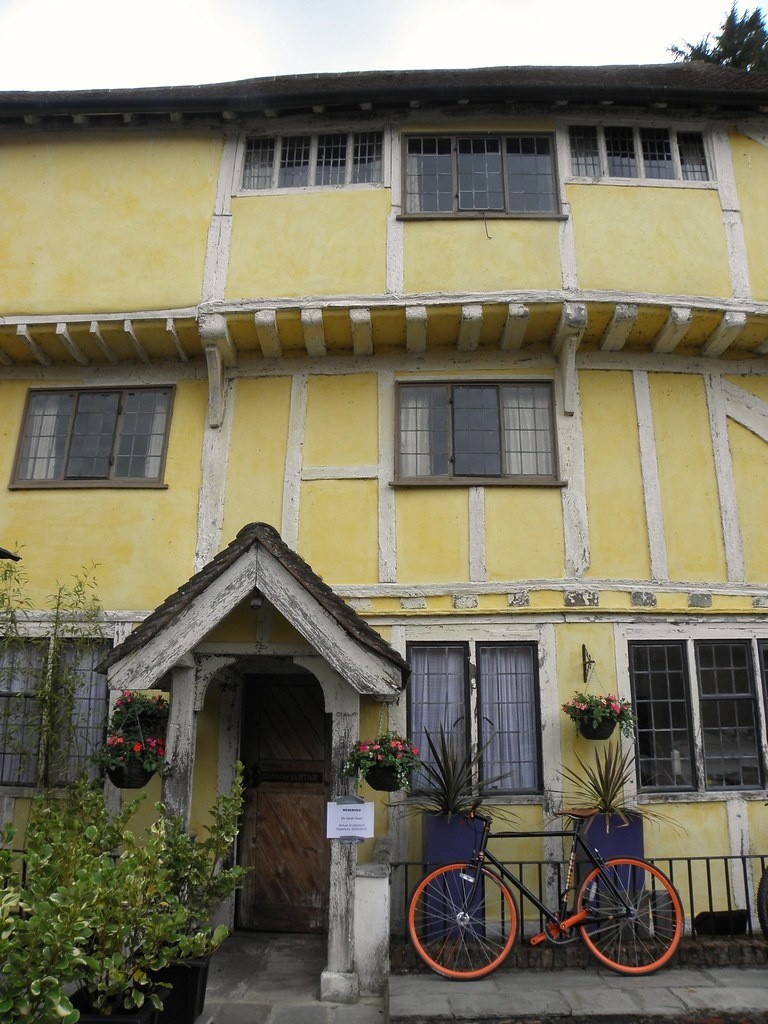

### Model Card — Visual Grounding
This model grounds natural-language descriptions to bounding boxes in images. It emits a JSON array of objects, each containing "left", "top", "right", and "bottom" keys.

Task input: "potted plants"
[
  {"left": 542, "top": 743, "right": 689, "bottom": 944},
  {"left": 0, "top": 759, "right": 255, "bottom": 1024},
  {"left": 401, "top": 699, "right": 524, "bottom": 946}
]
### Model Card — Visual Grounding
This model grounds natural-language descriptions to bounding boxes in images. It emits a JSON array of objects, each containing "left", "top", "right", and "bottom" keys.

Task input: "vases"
[
  {"left": 112, "top": 714, "right": 161, "bottom": 735},
  {"left": 571, "top": 716, "right": 618, "bottom": 740},
  {"left": 103, "top": 760, "right": 159, "bottom": 789},
  {"left": 361, "top": 764, "right": 407, "bottom": 791}
]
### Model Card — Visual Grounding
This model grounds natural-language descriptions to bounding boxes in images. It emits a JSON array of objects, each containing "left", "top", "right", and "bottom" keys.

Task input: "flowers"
[
  {"left": 91, "top": 687, "right": 172, "bottom": 770},
  {"left": 559, "top": 690, "right": 633, "bottom": 726},
  {"left": 341, "top": 724, "right": 432, "bottom": 773}
]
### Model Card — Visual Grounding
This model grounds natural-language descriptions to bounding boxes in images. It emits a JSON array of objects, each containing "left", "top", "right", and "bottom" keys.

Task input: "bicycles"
[{"left": 407, "top": 785, "right": 684, "bottom": 982}]
